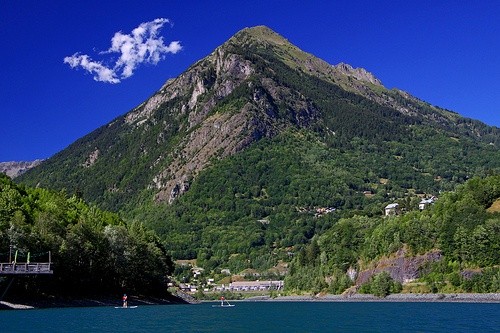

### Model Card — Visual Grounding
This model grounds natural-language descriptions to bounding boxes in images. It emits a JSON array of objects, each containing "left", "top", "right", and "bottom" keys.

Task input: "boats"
[
  {"left": 115, "top": 306, "right": 138, "bottom": 308},
  {"left": 212, "top": 297, "right": 235, "bottom": 307}
]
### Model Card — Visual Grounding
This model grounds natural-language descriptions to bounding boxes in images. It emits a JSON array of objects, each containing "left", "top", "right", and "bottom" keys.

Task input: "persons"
[{"left": 123, "top": 293, "right": 128, "bottom": 308}]
